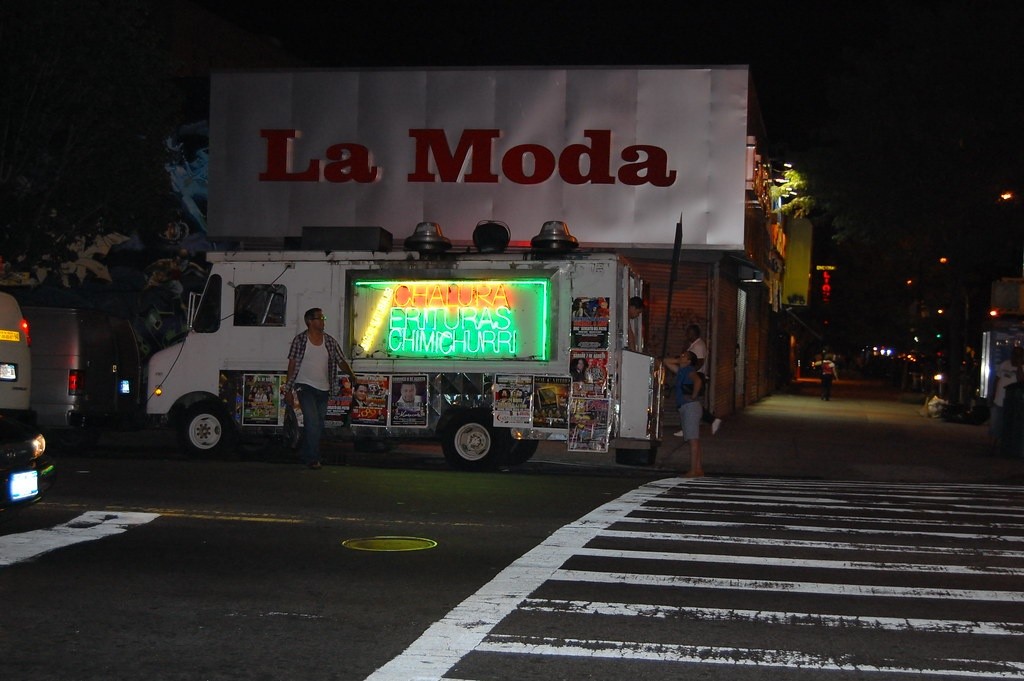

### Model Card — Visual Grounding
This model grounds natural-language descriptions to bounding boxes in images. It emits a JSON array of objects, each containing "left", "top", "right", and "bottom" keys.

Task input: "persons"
[
  {"left": 986, "top": 346, "right": 1024, "bottom": 457},
  {"left": 673, "top": 325, "right": 723, "bottom": 436},
  {"left": 572, "top": 358, "right": 585, "bottom": 381},
  {"left": 819, "top": 353, "right": 838, "bottom": 400},
  {"left": 397, "top": 381, "right": 421, "bottom": 411},
  {"left": 377, "top": 387, "right": 388, "bottom": 409},
  {"left": 664, "top": 350, "right": 705, "bottom": 476},
  {"left": 245, "top": 384, "right": 273, "bottom": 416},
  {"left": 352, "top": 384, "right": 367, "bottom": 409},
  {"left": 284, "top": 308, "right": 357, "bottom": 469},
  {"left": 627, "top": 296, "right": 644, "bottom": 352}
]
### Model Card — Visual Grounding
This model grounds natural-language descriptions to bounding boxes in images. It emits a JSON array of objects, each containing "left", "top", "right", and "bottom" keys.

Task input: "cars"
[{"left": 1, "top": 409, "right": 52, "bottom": 508}]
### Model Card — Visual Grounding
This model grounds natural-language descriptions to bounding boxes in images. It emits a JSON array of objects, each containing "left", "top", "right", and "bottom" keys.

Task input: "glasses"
[{"left": 310, "top": 316, "right": 327, "bottom": 320}]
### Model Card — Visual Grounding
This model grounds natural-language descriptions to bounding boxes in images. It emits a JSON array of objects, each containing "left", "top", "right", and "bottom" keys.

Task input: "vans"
[{"left": 20, "top": 306, "right": 142, "bottom": 455}]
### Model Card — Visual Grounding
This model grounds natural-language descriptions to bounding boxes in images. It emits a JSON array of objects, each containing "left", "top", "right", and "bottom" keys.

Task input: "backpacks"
[{"left": 821, "top": 361, "right": 833, "bottom": 374}]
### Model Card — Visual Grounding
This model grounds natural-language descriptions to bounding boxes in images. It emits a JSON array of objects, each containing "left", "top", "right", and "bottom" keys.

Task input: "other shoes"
[
  {"left": 311, "top": 459, "right": 322, "bottom": 469},
  {"left": 821, "top": 396, "right": 829, "bottom": 401},
  {"left": 672, "top": 430, "right": 684, "bottom": 437},
  {"left": 711, "top": 417, "right": 722, "bottom": 435},
  {"left": 684, "top": 469, "right": 705, "bottom": 478}
]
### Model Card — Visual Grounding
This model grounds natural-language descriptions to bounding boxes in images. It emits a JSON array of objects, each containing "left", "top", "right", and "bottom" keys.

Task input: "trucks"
[
  {"left": 0, "top": 292, "right": 57, "bottom": 514},
  {"left": 147, "top": 220, "right": 662, "bottom": 472}
]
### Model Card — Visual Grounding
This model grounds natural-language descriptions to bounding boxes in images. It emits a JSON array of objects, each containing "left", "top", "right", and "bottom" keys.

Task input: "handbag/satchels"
[{"left": 281, "top": 406, "right": 299, "bottom": 449}]
